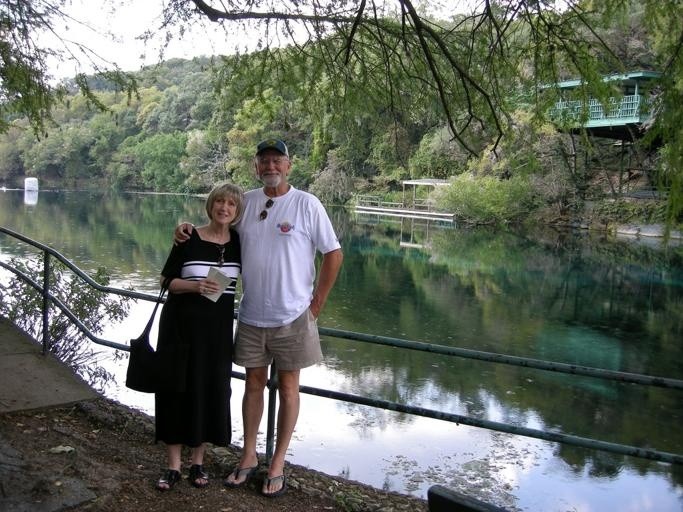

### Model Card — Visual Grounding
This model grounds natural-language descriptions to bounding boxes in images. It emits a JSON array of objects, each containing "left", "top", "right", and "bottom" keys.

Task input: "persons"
[
  {"left": 154, "top": 180, "right": 246, "bottom": 492},
  {"left": 172, "top": 139, "right": 344, "bottom": 499}
]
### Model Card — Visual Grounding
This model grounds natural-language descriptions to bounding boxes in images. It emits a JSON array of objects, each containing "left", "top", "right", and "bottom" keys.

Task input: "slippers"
[
  {"left": 262, "top": 468, "right": 287, "bottom": 497},
  {"left": 223, "top": 461, "right": 259, "bottom": 489}
]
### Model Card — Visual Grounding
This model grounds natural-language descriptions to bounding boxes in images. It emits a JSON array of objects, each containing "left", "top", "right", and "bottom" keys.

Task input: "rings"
[{"left": 204, "top": 287, "right": 207, "bottom": 292}]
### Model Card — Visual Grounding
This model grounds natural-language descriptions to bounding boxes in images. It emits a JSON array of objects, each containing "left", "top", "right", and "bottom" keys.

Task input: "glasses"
[
  {"left": 260, "top": 199, "right": 274, "bottom": 221},
  {"left": 217, "top": 245, "right": 225, "bottom": 267}
]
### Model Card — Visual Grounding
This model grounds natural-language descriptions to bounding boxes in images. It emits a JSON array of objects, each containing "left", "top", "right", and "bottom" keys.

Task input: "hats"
[{"left": 256, "top": 138, "right": 289, "bottom": 157}]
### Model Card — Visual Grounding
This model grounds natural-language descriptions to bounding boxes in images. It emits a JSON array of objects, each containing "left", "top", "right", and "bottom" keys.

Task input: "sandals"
[
  {"left": 156, "top": 468, "right": 183, "bottom": 492},
  {"left": 190, "top": 464, "right": 209, "bottom": 488}
]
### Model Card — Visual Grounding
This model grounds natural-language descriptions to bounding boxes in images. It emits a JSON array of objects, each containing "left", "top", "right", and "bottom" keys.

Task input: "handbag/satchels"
[{"left": 126, "top": 334, "right": 157, "bottom": 393}]
